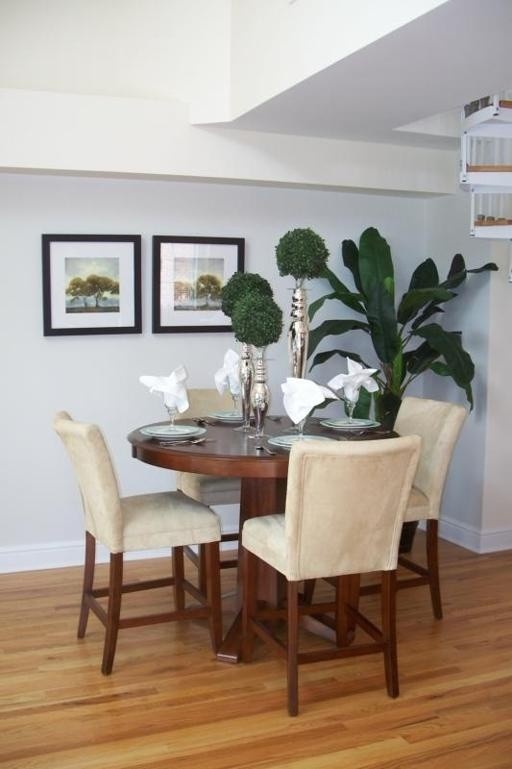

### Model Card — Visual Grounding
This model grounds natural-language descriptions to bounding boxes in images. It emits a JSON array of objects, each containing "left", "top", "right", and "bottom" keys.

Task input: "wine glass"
[
  {"left": 163, "top": 392, "right": 179, "bottom": 431},
  {"left": 298, "top": 420, "right": 306, "bottom": 440},
  {"left": 343, "top": 383, "right": 360, "bottom": 424},
  {"left": 227, "top": 377, "right": 241, "bottom": 416}
]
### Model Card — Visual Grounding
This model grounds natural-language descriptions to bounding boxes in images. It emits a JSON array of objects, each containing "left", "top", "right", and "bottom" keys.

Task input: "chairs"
[
  {"left": 173, "top": 389, "right": 242, "bottom": 596},
  {"left": 239, "top": 435, "right": 424, "bottom": 715},
  {"left": 348, "top": 395, "right": 468, "bottom": 642},
  {"left": 52, "top": 410, "right": 225, "bottom": 675}
]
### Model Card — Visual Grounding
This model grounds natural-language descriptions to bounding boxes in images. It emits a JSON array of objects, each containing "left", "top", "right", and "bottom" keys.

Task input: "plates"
[
  {"left": 320, "top": 419, "right": 381, "bottom": 431},
  {"left": 139, "top": 425, "right": 207, "bottom": 441},
  {"left": 267, "top": 434, "right": 337, "bottom": 449},
  {"left": 208, "top": 410, "right": 254, "bottom": 422}
]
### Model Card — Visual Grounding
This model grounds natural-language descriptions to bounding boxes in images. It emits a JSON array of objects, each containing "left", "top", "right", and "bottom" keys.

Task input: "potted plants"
[
  {"left": 231, "top": 291, "right": 284, "bottom": 439},
  {"left": 307, "top": 225, "right": 499, "bottom": 555},
  {"left": 221, "top": 271, "right": 274, "bottom": 434},
  {"left": 276, "top": 227, "right": 328, "bottom": 376}
]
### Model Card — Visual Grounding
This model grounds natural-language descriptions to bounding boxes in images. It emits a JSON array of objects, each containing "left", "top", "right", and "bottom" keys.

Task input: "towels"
[
  {"left": 139, "top": 364, "right": 189, "bottom": 414},
  {"left": 326, "top": 356, "right": 380, "bottom": 404},
  {"left": 280, "top": 377, "right": 341, "bottom": 425},
  {"left": 214, "top": 348, "right": 241, "bottom": 397}
]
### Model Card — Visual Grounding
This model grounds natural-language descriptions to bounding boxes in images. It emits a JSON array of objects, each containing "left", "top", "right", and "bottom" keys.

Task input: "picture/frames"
[
  {"left": 151, "top": 235, "right": 246, "bottom": 333},
  {"left": 41, "top": 234, "right": 142, "bottom": 336}
]
